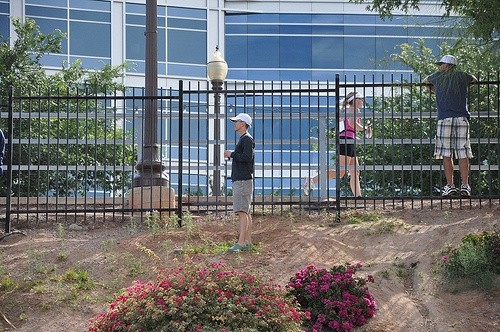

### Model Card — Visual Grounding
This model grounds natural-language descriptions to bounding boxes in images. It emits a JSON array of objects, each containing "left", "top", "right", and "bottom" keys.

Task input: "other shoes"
[
  {"left": 229, "top": 244, "right": 244, "bottom": 252},
  {"left": 242, "top": 244, "right": 251, "bottom": 251}
]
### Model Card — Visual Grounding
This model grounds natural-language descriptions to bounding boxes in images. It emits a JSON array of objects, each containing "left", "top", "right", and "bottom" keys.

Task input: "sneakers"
[
  {"left": 460, "top": 184, "right": 471, "bottom": 197},
  {"left": 442, "top": 184, "right": 457, "bottom": 196},
  {"left": 303, "top": 177, "right": 314, "bottom": 196}
]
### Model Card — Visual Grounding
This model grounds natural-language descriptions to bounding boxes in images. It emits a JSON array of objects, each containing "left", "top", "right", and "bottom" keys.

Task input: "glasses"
[{"left": 439, "top": 63, "right": 445, "bottom": 66}]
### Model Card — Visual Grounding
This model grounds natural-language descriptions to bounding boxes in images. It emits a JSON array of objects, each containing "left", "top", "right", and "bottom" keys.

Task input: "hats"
[
  {"left": 230, "top": 114, "right": 252, "bottom": 127},
  {"left": 347, "top": 92, "right": 366, "bottom": 104},
  {"left": 434, "top": 55, "right": 457, "bottom": 66}
]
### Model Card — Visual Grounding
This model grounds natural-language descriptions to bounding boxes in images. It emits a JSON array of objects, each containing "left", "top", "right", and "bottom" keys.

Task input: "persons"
[
  {"left": 222, "top": 112, "right": 256, "bottom": 254},
  {"left": 420, "top": 55, "right": 479, "bottom": 199},
  {"left": 304, "top": 91, "right": 364, "bottom": 202}
]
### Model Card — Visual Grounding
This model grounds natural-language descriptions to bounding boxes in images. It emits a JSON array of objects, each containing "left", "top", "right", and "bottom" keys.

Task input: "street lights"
[{"left": 206, "top": 44, "right": 229, "bottom": 197}]
